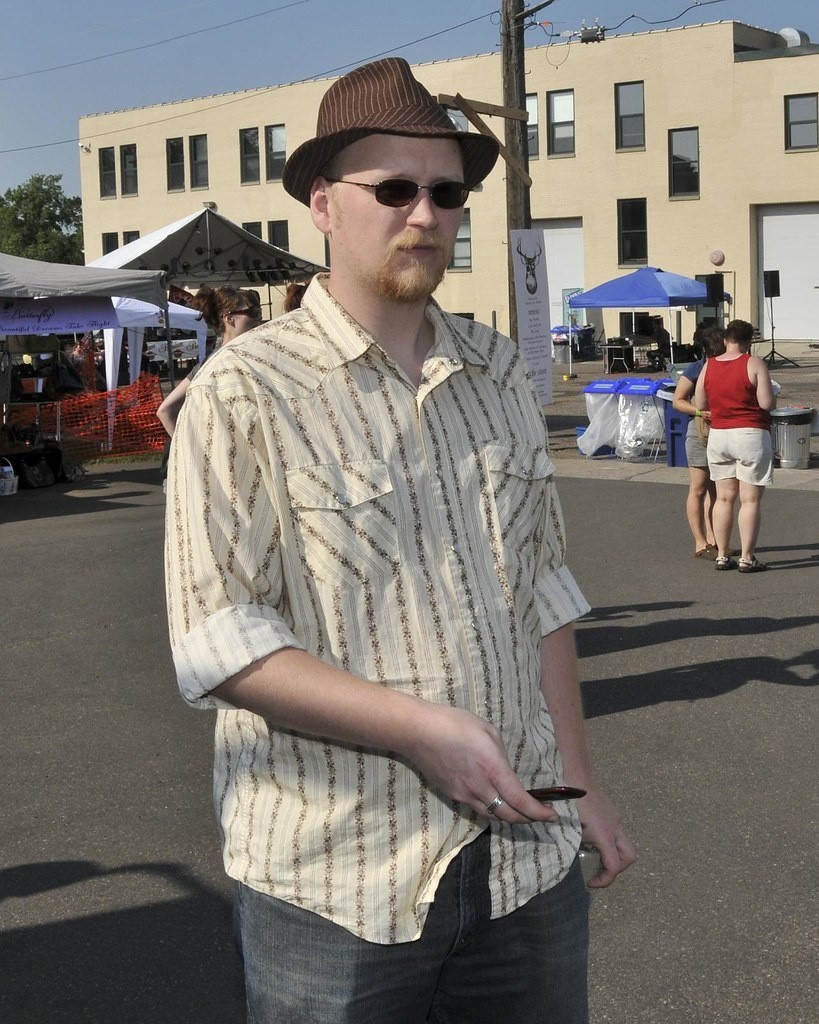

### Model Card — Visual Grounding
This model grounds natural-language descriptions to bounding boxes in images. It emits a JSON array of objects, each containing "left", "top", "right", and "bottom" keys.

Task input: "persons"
[
  {"left": 695, "top": 320, "right": 781, "bottom": 573},
  {"left": 284, "top": 280, "right": 312, "bottom": 313},
  {"left": 693, "top": 323, "right": 707, "bottom": 360},
  {"left": 155, "top": 282, "right": 264, "bottom": 438},
  {"left": 6, "top": 334, "right": 148, "bottom": 402},
  {"left": 636, "top": 318, "right": 670, "bottom": 370},
  {"left": 163, "top": 57, "right": 635, "bottom": 1023},
  {"left": 672, "top": 326, "right": 739, "bottom": 561}
]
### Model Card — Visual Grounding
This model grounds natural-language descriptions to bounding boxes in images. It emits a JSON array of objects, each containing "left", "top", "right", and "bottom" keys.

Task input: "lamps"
[{"left": 202, "top": 201, "right": 215, "bottom": 207}]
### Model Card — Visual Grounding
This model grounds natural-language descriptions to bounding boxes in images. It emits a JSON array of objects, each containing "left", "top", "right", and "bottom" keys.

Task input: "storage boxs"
[{"left": 576, "top": 426, "right": 615, "bottom": 455}]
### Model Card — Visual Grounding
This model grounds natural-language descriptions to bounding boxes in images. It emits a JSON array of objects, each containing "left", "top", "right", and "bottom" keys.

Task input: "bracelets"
[
  {"left": 696, "top": 409, "right": 701, "bottom": 417},
  {"left": 486, "top": 794, "right": 502, "bottom": 814}
]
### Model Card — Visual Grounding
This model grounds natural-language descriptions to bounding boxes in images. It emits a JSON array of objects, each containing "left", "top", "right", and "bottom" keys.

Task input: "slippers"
[
  {"left": 712, "top": 542, "right": 736, "bottom": 556},
  {"left": 695, "top": 546, "right": 719, "bottom": 561}
]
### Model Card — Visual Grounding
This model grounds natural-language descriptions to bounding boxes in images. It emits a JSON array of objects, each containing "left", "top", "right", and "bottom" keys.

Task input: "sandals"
[
  {"left": 715, "top": 556, "right": 736, "bottom": 571},
  {"left": 739, "top": 555, "right": 767, "bottom": 573}
]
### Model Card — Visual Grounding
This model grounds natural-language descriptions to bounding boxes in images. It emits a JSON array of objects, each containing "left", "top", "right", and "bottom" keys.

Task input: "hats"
[{"left": 282, "top": 57, "right": 500, "bottom": 206}]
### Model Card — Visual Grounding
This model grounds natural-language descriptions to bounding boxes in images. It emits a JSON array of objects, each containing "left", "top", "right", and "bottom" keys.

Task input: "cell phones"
[{"left": 526, "top": 786, "right": 587, "bottom": 801}]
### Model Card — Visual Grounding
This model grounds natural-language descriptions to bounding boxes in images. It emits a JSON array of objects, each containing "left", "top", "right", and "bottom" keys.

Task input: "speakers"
[
  {"left": 695, "top": 273, "right": 724, "bottom": 307},
  {"left": 764, "top": 271, "right": 780, "bottom": 297}
]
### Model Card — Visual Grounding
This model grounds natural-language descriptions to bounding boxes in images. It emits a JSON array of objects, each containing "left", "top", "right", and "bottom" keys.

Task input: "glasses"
[
  {"left": 322, "top": 178, "right": 474, "bottom": 210},
  {"left": 219, "top": 305, "right": 263, "bottom": 319}
]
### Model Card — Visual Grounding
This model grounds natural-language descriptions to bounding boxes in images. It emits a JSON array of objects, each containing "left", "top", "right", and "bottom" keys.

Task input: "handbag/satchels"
[
  {"left": 696, "top": 416, "right": 710, "bottom": 448},
  {"left": 23, "top": 460, "right": 55, "bottom": 488}
]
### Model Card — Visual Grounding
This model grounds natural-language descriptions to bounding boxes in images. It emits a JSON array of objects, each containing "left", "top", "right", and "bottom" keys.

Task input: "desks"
[{"left": 599, "top": 343, "right": 634, "bottom": 375}]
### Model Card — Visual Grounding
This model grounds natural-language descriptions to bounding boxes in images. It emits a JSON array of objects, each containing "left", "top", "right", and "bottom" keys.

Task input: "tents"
[
  {"left": 0, "top": 252, "right": 175, "bottom": 391},
  {"left": 86, "top": 209, "right": 330, "bottom": 387},
  {"left": 568, "top": 266, "right": 731, "bottom": 374},
  {"left": 102, "top": 296, "right": 208, "bottom": 451}
]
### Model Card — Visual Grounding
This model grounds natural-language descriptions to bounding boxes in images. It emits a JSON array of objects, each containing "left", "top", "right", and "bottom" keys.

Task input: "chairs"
[{"left": 646, "top": 340, "right": 678, "bottom": 371}]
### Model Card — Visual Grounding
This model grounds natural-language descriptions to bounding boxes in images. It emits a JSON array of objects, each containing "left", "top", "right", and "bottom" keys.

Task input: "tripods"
[{"left": 762, "top": 296, "right": 800, "bottom": 367}]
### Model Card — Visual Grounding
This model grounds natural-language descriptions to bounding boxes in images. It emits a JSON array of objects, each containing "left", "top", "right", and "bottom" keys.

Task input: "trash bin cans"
[
  {"left": 575, "top": 376, "right": 696, "bottom": 468},
  {"left": 769, "top": 403, "right": 816, "bottom": 470},
  {"left": 607, "top": 336, "right": 626, "bottom": 373},
  {"left": 549, "top": 324, "right": 596, "bottom": 364}
]
